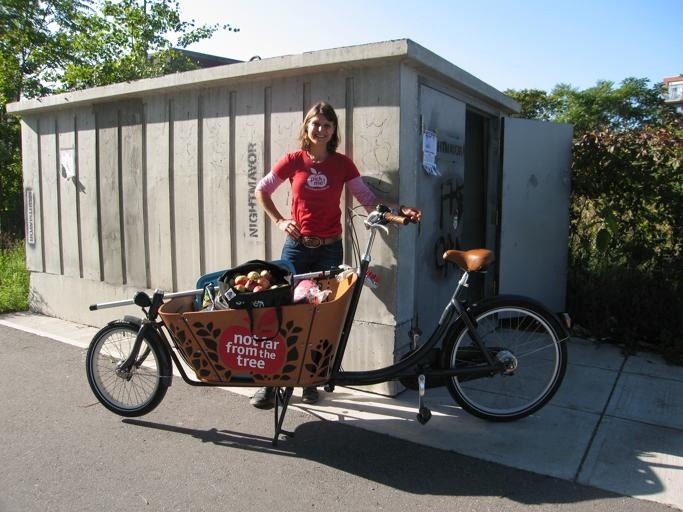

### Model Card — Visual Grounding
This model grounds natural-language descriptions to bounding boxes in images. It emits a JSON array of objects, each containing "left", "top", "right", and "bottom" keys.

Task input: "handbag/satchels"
[{"left": 213, "top": 260, "right": 294, "bottom": 310}]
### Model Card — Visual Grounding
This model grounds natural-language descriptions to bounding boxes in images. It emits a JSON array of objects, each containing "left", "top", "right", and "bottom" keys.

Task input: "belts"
[{"left": 300, "top": 236, "right": 342, "bottom": 250}]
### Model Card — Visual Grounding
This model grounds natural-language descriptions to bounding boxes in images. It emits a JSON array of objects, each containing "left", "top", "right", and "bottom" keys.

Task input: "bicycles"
[{"left": 86, "top": 206, "right": 571, "bottom": 419}]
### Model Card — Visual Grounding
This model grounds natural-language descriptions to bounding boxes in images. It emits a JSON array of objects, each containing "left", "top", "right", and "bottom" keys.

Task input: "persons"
[{"left": 251, "top": 101, "right": 423, "bottom": 409}]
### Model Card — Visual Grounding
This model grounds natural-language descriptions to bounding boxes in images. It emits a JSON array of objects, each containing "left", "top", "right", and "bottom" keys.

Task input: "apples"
[
  {"left": 232, "top": 269, "right": 273, "bottom": 293},
  {"left": 219, "top": 309, "right": 287, "bottom": 374}
]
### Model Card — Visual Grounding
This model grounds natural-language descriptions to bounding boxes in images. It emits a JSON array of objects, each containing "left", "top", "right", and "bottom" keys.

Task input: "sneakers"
[
  {"left": 301, "top": 386, "right": 318, "bottom": 404},
  {"left": 249, "top": 387, "right": 279, "bottom": 409}
]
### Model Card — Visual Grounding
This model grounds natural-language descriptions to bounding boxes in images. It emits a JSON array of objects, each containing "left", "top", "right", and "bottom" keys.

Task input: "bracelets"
[{"left": 399, "top": 205, "right": 406, "bottom": 216}]
